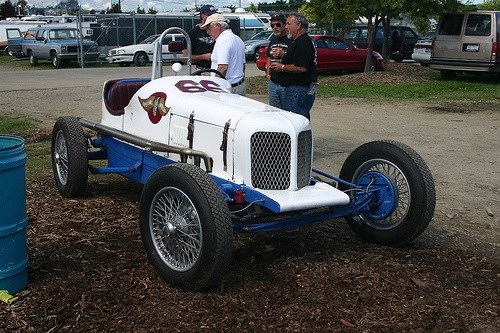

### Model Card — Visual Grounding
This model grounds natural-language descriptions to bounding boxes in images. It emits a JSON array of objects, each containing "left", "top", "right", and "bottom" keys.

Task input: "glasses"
[{"left": 270, "top": 23, "right": 285, "bottom": 27}]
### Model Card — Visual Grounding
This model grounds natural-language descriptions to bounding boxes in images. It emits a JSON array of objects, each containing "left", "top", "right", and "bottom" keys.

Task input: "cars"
[
  {"left": 255, "top": 34, "right": 385, "bottom": 75},
  {"left": 411, "top": 31, "right": 436, "bottom": 67},
  {"left": 243, "top": 30, "right": 275, "bottom": 62},
  {"left": 105, "top": 33, "right": 188, "bottom": 67}
]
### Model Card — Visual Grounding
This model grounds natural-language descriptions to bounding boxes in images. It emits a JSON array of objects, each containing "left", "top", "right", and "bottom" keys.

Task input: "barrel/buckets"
[{"left": 0, "top": 134, "right": 28, "bottom": 295}]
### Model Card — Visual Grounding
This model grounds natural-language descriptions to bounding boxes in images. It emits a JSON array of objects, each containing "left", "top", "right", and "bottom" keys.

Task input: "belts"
[{"left": 231, "top": 77, "right": 245, "bottom": 87}]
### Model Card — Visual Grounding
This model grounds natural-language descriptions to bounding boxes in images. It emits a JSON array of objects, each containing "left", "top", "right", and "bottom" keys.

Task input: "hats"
[
  {"left": 200, "top": 12, "right": 225, "bottom": 28},
  {"left": 269, "top": 12, "right": 287, "bottom": 20},
  {"left": 200, "top": 4, "right": 221, "bottom": 16}
]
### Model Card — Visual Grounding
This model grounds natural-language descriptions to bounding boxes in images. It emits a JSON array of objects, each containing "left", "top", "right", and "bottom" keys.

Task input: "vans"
[
  {"left": 337, "top": 25, "right": 422, "bottom": 63},
  {"left": 428, "top": 10, "right": 500, "bottom": 80}
]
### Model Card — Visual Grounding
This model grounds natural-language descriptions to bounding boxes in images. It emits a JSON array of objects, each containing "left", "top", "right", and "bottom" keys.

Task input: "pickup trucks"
[{"left": 5, "top": 24, "right": 100, "bottom": 69}]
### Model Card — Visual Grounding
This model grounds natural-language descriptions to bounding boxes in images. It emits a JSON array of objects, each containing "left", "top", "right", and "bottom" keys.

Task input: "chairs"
[
  {"left": 392, "top": 31, "right": 401, "bottom": 40},
  {"left": 317, "top": 41, "right": 328, "bottom": 48},
  {"left": 476, "top": 20, "right": 491, "bottom": 32},
  {"left": 103, "top": 77, "right": 151, "bottom": 116}
]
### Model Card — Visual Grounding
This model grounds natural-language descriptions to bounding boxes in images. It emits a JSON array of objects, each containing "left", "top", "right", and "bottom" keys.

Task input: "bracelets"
[
  {"left": 282, "top": 65, "right": 285, "bottom": 72},
  {"left": 284, "top": 50, "right": 287, "bottom": 53}
]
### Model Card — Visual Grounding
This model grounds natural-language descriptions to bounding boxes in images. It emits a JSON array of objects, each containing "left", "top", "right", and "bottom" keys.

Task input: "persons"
[
  {"left": 270, "top": 13, "right": 317, "bottom": 121},
  {"left": 200, "top": 13, "right": 247, "bottom": 96},
  {"left": 183, "top": 4, "right": 216, "bottom": 76},
  {"left": 266, "top": 12, "right": 289, "bottom": 108}
]
